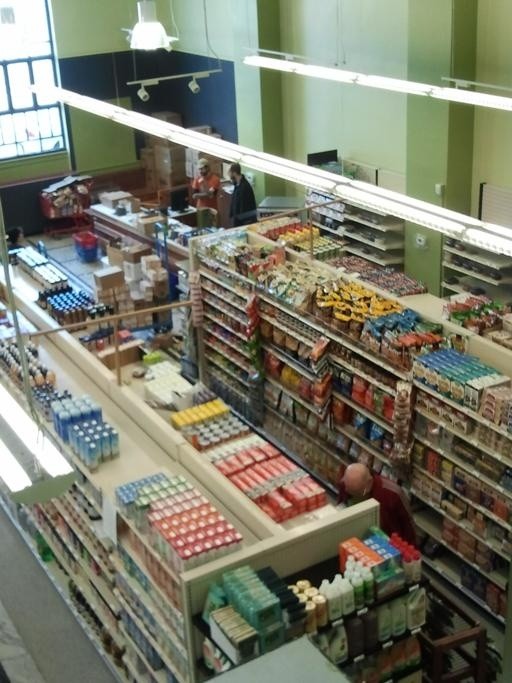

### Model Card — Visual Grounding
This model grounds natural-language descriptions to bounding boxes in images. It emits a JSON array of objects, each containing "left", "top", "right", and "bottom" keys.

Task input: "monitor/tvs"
[{"left": 171, "top": 188, "right": 188, "bottom": 213}]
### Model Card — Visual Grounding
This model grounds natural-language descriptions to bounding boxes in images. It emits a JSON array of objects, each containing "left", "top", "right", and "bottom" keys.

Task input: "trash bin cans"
[{"left": 72, "top": 231, "right": 98, "bottom": 262}]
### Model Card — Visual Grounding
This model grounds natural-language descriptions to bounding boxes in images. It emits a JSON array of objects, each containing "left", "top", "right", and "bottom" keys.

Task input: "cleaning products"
[{"left": 307, "top": 621, "right": 350, "bottom": 665}]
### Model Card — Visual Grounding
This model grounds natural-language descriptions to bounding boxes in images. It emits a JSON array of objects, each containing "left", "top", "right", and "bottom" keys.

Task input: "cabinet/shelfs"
[{"left": 2, "top": 188, "right": 511, "bottom": 683}]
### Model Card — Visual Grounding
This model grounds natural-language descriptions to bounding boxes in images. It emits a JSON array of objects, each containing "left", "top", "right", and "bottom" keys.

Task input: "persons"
[
  {"left": 191, "top": 158, "right": 219, "bottom": 226},
  {"left": 228, "top": 163, "right": 258, "bottom": 226},
  {"left": 337, "top": 462, "right": 418, "bottom": 552},
  {"left": 5, "top": 226, "right": 25, "bottom": 256}
]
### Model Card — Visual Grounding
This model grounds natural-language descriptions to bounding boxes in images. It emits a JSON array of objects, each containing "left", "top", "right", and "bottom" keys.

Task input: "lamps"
[
  {"left": 29, "top": 81, "right": 512, "bottom": 258},
  {"left": 137, "top": 89, "right": 149, "bottom": 101},
  {"left": 130, "top": 0, "right": 170, "bottom": 50},
  {"left": 241, "top": 53, "right": 511, "bottom": 114},
  {"left": 188, "top": 80, "right": 200, "bottom": 94}
]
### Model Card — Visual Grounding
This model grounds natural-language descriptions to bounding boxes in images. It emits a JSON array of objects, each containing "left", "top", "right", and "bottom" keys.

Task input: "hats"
[{"left": 195, "top": 158, "right": 209, "bottom": 169}]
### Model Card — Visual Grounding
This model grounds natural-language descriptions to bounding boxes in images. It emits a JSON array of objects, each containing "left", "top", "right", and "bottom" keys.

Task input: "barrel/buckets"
[{"left": 406, "top": 583, "right": 433, "bottom": 633}]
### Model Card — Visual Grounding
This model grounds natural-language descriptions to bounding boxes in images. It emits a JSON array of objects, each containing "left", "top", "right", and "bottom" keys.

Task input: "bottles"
[
  {"left": 31, "top": 264, "right": 118, "bottom": 335},
  {"left": 4, "top": 342, "right": 70, "bottom": 422},
  {"left": 343, "top": 555, "right": 375, "bottom": 609}
]
